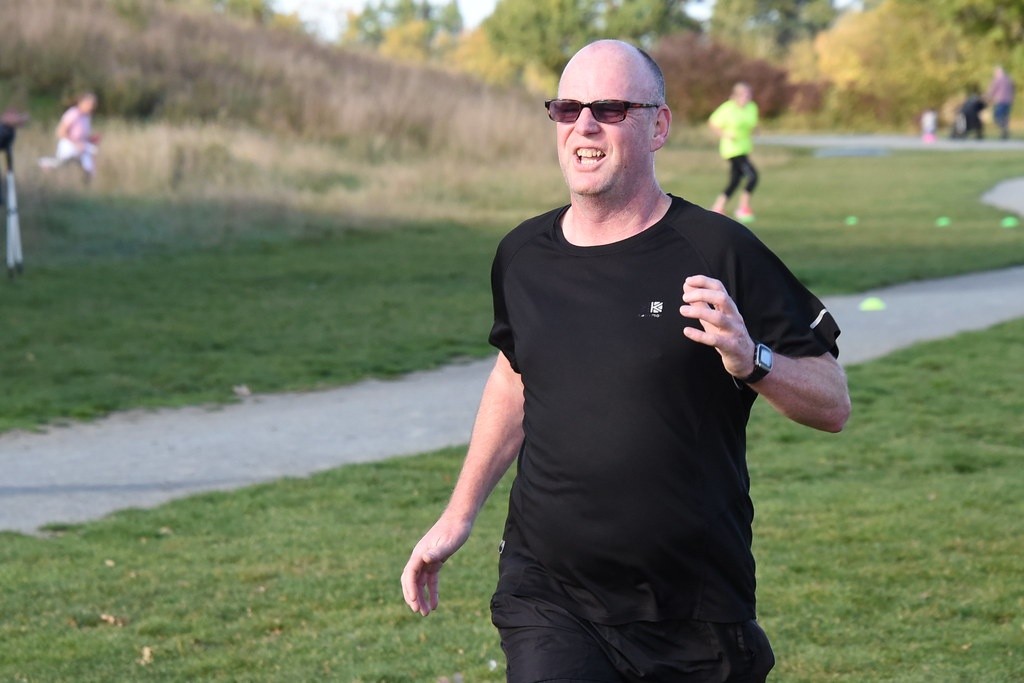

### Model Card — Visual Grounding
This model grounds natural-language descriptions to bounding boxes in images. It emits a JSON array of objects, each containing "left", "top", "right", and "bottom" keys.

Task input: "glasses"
[{"left": 545, "top": 100, "right": 658, "bottom": 122}]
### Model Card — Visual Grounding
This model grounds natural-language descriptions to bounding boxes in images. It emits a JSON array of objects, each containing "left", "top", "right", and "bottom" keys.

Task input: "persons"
[
  {"left": 38, "top": 93, "right": 103, "bottom": 195},
  {"left": 705, "top": 80, "right": 762, "bottom": 220},
  {"left": 919, "top": 65, "right": 1016, "bottom": 142},
  {"left": 401, "top": 38, "right": 852, "bottom": 683}
]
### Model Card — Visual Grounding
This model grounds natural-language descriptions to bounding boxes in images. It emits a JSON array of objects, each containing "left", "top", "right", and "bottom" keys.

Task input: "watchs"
[{"left": 731, "top": 338, "right": 773, "bottom": 392}]
